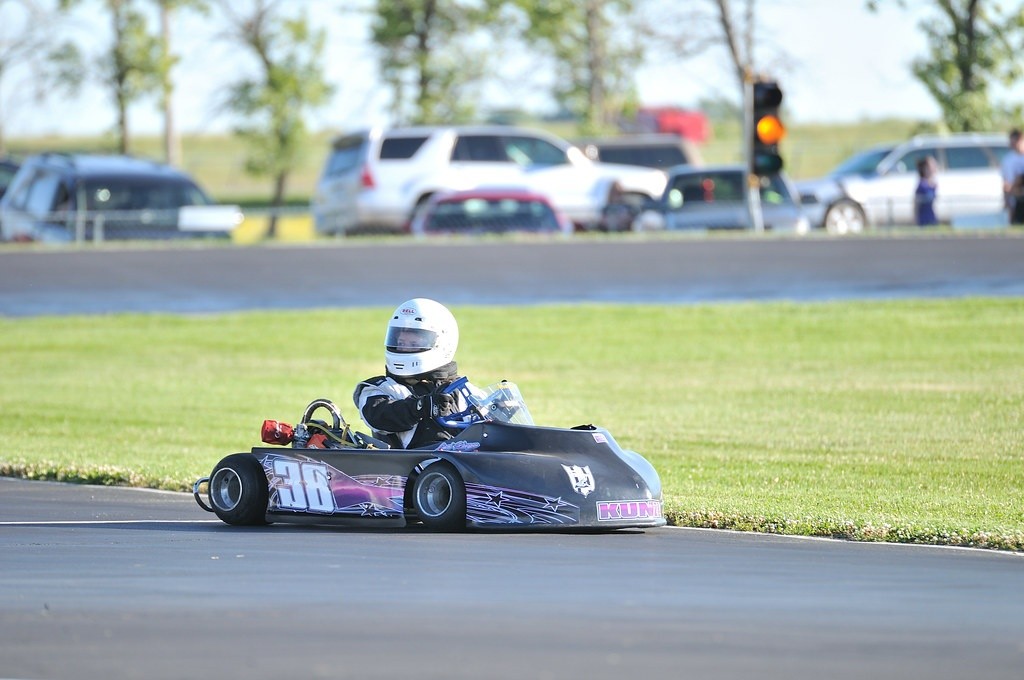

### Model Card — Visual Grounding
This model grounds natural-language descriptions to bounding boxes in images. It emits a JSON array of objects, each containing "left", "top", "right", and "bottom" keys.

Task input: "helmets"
[{"left": 383, "top": 298, "right": 459, "bottom": 376}]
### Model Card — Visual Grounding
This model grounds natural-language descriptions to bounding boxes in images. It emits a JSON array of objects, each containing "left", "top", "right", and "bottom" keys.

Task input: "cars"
[
  {"left": 406, "top": 188, "right": 572, "bottom": 235},
  {"left": 627, "top": 106, "right": 708, "bottom": 141},
  {"left": 661, "top": 163, "right": 810, "bottom": 229}
]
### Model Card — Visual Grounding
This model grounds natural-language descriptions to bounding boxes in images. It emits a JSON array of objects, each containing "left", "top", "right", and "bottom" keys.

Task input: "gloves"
[{"left": 411, "top": 381, "right": 454, "bottom": 418}]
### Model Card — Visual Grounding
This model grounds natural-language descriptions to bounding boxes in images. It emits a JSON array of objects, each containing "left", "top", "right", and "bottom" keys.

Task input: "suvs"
[
  {"left": 797, "top": 133, "right": 1012, "bottom": 235},
  {"left": 315, "top": 124, "right": 700, "bottom": 234},
  {"left": 0, "top": 152, "right": 233, "bottom": 244}
]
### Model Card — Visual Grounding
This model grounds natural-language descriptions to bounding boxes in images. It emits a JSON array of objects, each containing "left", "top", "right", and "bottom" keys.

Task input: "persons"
[
  {"left": 914, "top": 155, "right": 939, "bottom": 226},
  {"left": 353, "top": 298, "right": 519, "bottom": 449},
  {"left": 1001, "top": 131, "right": 1024, "bottom": 225}
]
